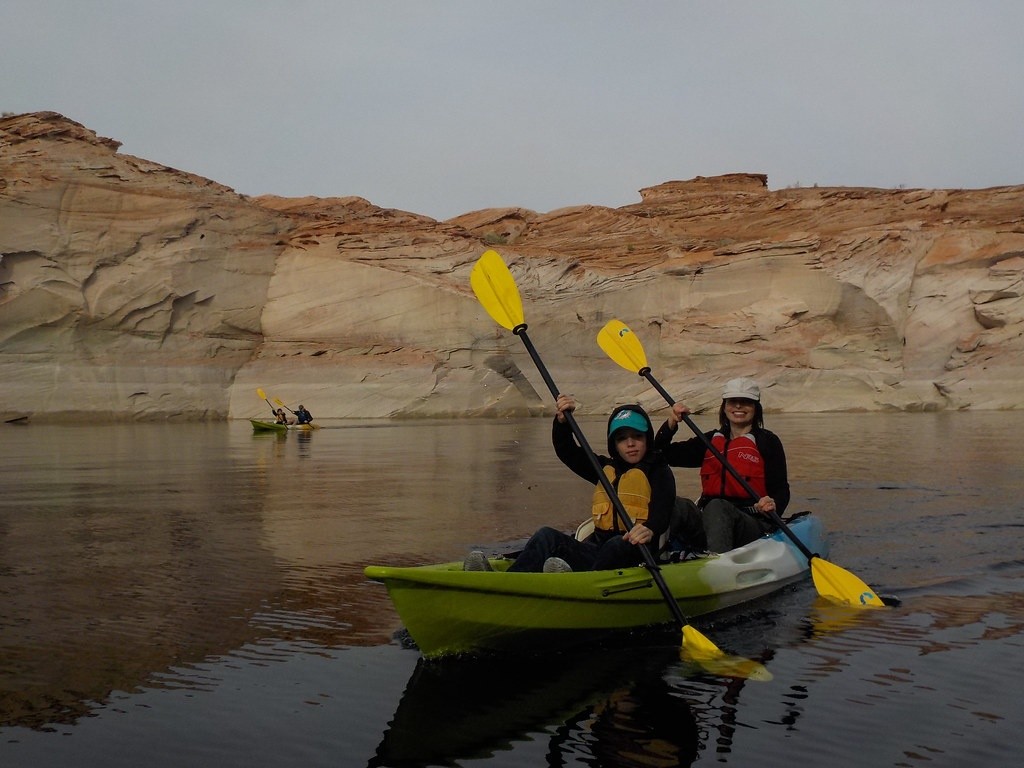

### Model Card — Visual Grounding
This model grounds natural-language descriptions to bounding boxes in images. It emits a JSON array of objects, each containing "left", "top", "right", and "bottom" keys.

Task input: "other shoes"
[
  {"left": 463, "top": 550, "right": 493, "bottom": 571},
  {"left": 542, "top": 557, "right": 573, "bottom": 573}
]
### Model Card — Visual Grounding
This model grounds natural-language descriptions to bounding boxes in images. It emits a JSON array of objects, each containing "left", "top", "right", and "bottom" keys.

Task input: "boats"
[
  {"left": 364, "top": 509, "right": 830, "bottom": 662},
  {"left": 249, "top": 416, "right": 320, "bottom": 431}
]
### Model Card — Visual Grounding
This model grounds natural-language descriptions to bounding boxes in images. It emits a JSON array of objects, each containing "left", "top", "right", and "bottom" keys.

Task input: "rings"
[{"left": 770, "top": 503, "right": 772, "bottom": 505}]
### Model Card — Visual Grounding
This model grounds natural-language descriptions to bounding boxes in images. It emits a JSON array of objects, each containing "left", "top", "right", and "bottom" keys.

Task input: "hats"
[
  {"left": 299, "top": 405, "right": 304, "bottom": 410},
  {"left": 608, "top": 410, "right": 648, "bottom": 437},
  {"left": 722, "top": 377, "right": 760, "bottom": 401}
]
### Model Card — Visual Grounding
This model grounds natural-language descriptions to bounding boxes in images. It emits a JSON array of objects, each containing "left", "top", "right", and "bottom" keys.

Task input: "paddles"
[
  {"left": 274, "top": 399, "right": 315, "bottom": 429},
  {"left": 257, "top": 388, "right": 288, "bottom": 429},
  {"left": 595, "top": 316, "right": 884, "bottom": 608},
  {"left": 468, "top": 248, "right": 722, "bottom": 661}
]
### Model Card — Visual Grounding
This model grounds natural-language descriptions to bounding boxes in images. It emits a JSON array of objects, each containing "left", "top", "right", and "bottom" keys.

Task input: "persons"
[
  {"left": 293, "top": 405, "right": 313, "bottom": 425},
  {"left": 463, "top": 394, "right": 677, "bottom": 574},
  {"left": 654, "top": 377, "right": 790, "bottom": 554},
  {"left": 272, "top": 408, "right": 287, "bottom": 425}
]
